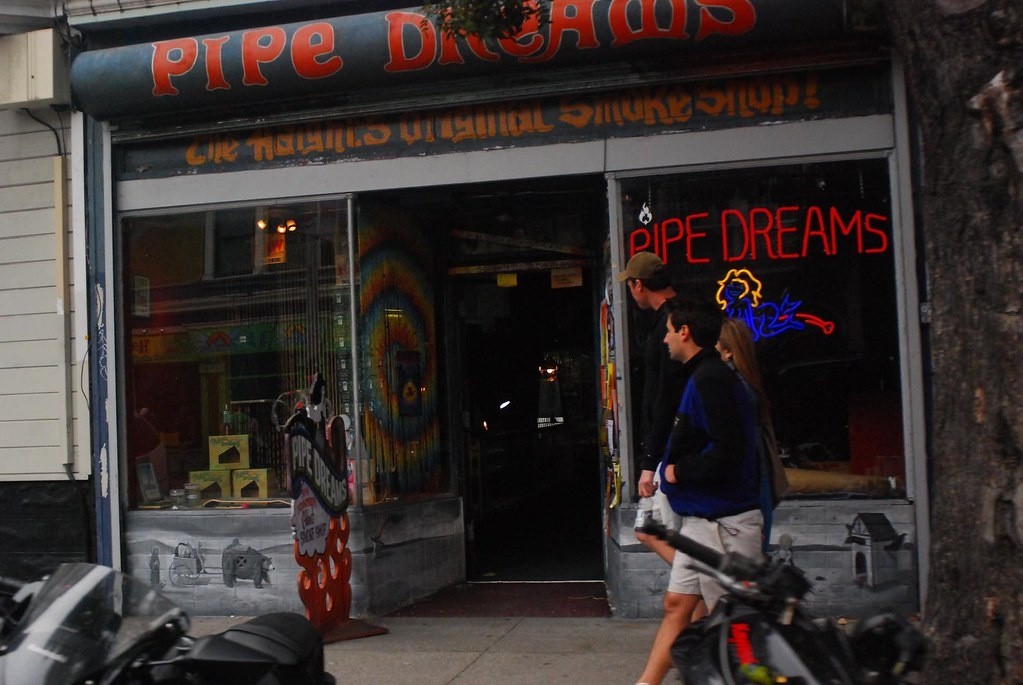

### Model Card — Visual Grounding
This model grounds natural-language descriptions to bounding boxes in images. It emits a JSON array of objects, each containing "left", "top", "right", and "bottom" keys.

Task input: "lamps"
[{"left": 256, "top": 214, "right": 297, "bottom": 233}]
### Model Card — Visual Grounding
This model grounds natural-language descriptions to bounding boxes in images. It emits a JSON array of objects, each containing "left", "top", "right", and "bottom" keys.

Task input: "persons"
[{"left": 616, "top": 252, "right": 789, "bottom": 685}]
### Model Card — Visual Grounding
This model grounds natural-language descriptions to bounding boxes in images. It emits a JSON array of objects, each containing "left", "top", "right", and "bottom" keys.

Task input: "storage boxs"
[
  {"left": 347, "top": 458, "right": 376, "bottom": 506},
  {"left": 188, "top": 434, "right": 279, "bottom": 507}
]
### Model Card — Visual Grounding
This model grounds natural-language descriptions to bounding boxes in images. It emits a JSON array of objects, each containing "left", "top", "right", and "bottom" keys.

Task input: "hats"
[{"left": 616, "top": 251, "right": 663, "bottom": 282}]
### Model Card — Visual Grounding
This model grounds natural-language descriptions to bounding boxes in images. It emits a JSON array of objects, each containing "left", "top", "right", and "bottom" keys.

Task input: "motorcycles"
[
  {"left": 1, "top": 561, "right": 336, "bottom": 685},
  {"left": 642, "top": 513, "right": 930, "bottom": 685}
]
